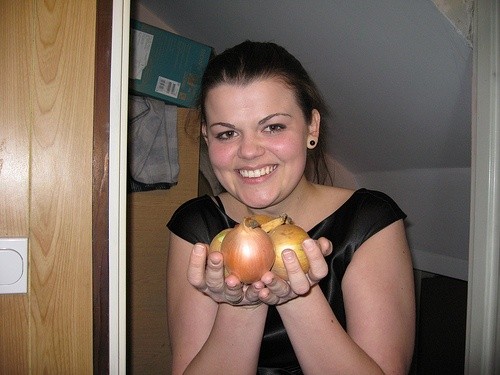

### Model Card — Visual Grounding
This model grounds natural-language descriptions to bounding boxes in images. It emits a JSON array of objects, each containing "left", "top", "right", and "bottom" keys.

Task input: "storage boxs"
[{"left": 128, "top": 18, "right": 216, "bottom": 110}]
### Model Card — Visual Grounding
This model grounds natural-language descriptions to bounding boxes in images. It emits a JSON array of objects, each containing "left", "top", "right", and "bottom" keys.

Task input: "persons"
[{"left": 166, "top": 39, "right": 416, "bottom": 374}]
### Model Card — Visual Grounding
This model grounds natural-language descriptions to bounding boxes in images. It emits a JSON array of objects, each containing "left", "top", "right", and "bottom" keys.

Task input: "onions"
[{"left": 208, "top": 214, "right": 310, "bottom": 285}]
